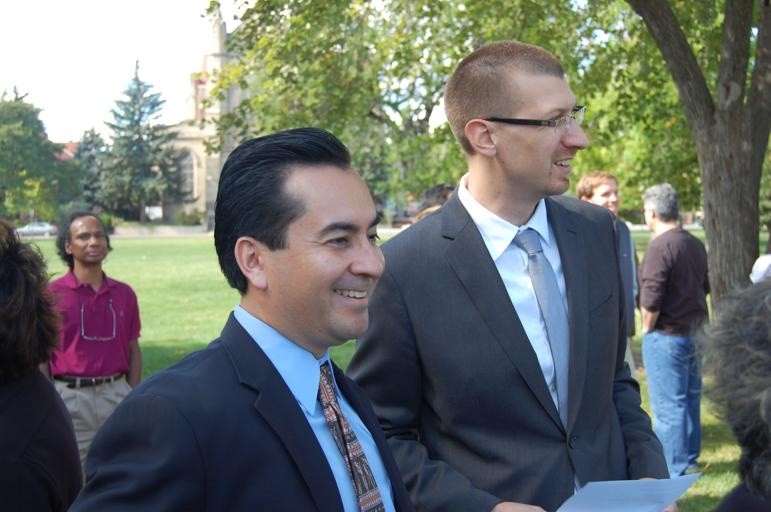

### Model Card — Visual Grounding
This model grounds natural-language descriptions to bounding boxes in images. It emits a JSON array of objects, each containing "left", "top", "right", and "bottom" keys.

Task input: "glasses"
[
  {"left": 483, "top": 105, "right": 587, "bottom": 127},
  {"left": 81, "top": 303, "right": 116, "bottom": 340}
]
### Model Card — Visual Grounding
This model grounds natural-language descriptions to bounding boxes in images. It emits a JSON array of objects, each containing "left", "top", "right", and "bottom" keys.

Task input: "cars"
[
  {"left": 391, "top": 204, "right": 441, "bottom": 226},
  {"left": 14, "top": 221, "right": 59, "bottom": 238},
  {"left": 695, "top": 219, "right": 704, "bottom": 228}
]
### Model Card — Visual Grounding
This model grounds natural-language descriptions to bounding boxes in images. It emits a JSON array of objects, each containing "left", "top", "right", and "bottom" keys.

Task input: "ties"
[
  {"left": 318, "top": 362, "right": 384, "bottom": 512},
  {"left": 513, "top": 230, "right": 569, "bottom": 432}
]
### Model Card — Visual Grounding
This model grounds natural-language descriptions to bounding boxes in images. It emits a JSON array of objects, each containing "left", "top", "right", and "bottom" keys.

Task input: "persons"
[
  {"left": 639, "top": 184, "right": 710, "bottom": 479},
  {"left": 345, "top": 41, "right": 678, "bottom": 512},
  {"left": 577, "top": 173, "right": 640, "bottom": 382},
  {"left": 412, "top": 185, "right": 455, "bottom": 224},
  {"left": 689, "top": 277, "right": 771, "bottom": 512},
  {"left": 67, "top": 127, "right": 409, "bottom": 512},
  {"left": 0, "top": 220, "right": 82, "bottom": 512},
  {"left": 38, "top": 212, "right": 141, "bottom": 487}
]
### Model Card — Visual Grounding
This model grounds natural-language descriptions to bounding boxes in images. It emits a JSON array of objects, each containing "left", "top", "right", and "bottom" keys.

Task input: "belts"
[{"left": 53, "top": 372, "right": 123, "bottom": 388}]
